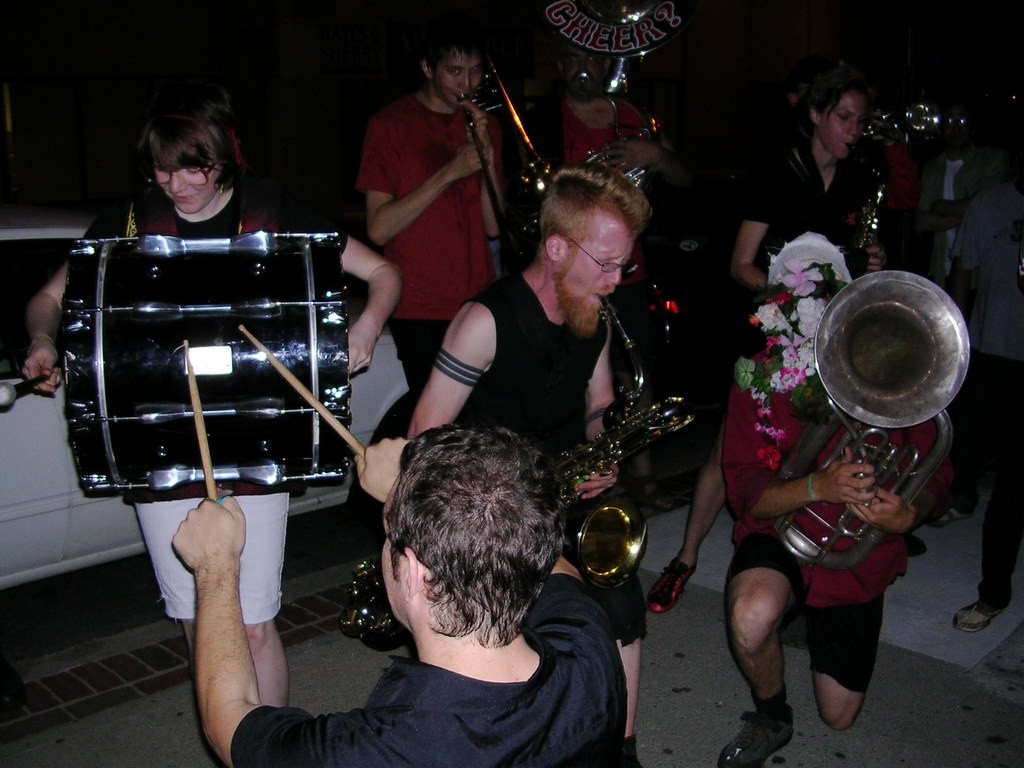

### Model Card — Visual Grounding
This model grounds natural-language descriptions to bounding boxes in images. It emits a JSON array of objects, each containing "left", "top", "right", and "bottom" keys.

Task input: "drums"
[{"left": 57, "top": 226, "right": 355, "bottom": 505}]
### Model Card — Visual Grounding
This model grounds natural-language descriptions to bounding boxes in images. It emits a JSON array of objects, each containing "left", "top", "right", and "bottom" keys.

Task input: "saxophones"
[
  {"left": 847, "top": 139, "right": 885, "bottom": 273},
  {"left": 338, "top": 294, "right": 702, "bottom": 653}
]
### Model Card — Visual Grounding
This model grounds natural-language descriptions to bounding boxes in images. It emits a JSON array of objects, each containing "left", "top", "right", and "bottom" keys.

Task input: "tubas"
[{"left": 761, "top": 269, "right": 974, "bottom": 575}]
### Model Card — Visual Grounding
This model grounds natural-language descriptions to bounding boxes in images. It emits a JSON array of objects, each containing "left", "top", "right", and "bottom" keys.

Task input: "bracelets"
[
  {"left": 806, "top": 472, "right": 816, "bottom": 505},
  {"left": 27, "top": 334, "right": 56, "bottom": 357}
]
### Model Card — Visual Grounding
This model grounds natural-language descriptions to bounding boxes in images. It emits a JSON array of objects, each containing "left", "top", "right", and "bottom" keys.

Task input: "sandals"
[{"left": 633, "top": 475, "right": 673, "bottom": 512}]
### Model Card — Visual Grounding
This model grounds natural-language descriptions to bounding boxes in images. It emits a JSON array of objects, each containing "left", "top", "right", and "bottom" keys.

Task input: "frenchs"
[{"left": 543, "top": 1, "right": 692, "bottom": 201}]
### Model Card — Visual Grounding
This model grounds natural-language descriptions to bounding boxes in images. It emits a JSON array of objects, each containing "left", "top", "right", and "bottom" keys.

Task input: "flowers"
[{"left": 737, "top": 257, "right": 855, "bottom": 473}]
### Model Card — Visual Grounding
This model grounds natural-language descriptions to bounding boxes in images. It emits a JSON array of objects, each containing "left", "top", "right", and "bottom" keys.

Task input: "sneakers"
[
  {"left": 953, "top": 598, "right": 1002, "bottom": 633},
  {"left": 647, "top": 555, "right": 699, "bottom": 613},
  {"left": 781, "top": 620, "right": 808, "bottom": 649},
  {"left": 717, "top": 705, "right": 794, "bottom": 768},
  {"left": 928, "top": 508, "right": 972, "bottom": 527}
]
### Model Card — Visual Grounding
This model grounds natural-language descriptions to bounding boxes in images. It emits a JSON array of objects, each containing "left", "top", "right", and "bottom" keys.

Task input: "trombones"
[{"left": 464, "top": 58, "right": 566, "bottom": 261}]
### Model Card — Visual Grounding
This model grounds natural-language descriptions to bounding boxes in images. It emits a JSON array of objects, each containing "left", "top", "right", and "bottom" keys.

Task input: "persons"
[
  {"left": 172, "top": 421, "right": 628, "bottom": 768},
  {"left": 717, "top": 229, "right": 952, "bottom": 768},
  {"left": 354, "top": 13, "right": 505, "bottom": 389},
  {"left": 22, "top": 92, "right": 403, "bottom": 708},
  {"left": 407, "top": 162, "right": 653, "bottom": 768},
  {"left": 647, "top": 68, "right": 1024, "bottom": 633},
  {"left": 518, "top": 41, "right": 691, "bottom": 291}
]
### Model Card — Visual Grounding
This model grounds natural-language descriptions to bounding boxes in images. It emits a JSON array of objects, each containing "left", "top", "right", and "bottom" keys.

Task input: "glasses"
[
  {"left": 569, "top": 237, "right": 639, "bottom": 274},
  {"left": 153, "top": 163, "right": 215, "bottom": 186},
  {"left": 584, "top": 55, "right": 596, "bottom": 66}
]
